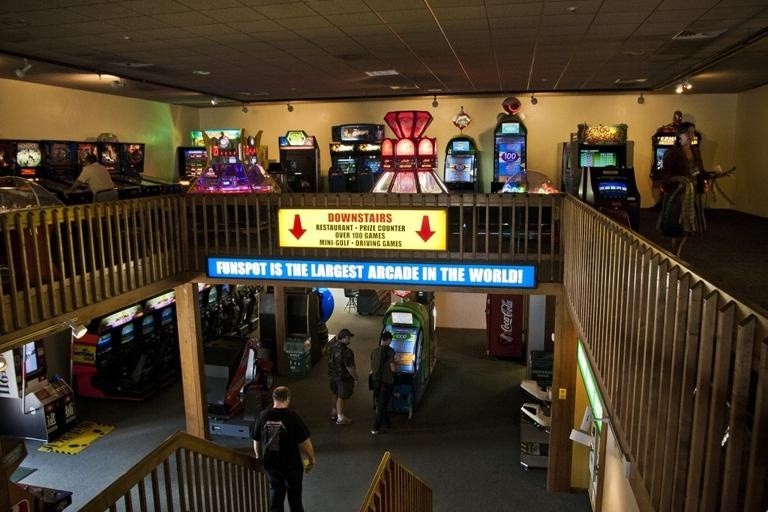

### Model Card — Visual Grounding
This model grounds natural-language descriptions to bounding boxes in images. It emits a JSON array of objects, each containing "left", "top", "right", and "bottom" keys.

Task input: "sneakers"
[{"left": 330, "top": 413, "right": 352, "bottom": 425}]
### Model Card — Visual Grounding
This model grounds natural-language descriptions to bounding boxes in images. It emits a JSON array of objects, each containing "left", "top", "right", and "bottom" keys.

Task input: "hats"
[{"left": 338, "top": 328, "right": 354, "bottom": 339}]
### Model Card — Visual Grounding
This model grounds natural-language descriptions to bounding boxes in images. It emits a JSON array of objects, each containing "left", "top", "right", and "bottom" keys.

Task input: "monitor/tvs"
[
  {"left": 599, "top": 180, "right": 629, "bottom": 200},
  {"left": 579, "top": 149, "right": 620, "bottom": 168},
  {"left": 656, "top": 148, "right": 669, "bottom": 171},
  {"left": 23, "top": 342, "right": 39, "bottom": 374},
  {"left": 97, "top": 283, "right": 231, "bottom": 357}
]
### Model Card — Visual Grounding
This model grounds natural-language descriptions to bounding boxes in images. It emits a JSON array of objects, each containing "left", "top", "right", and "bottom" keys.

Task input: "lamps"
[
  {"left": 241, "top": 104, "right": 248, "bottom": 112},
  {"left": 637, "top": 96, "right": 647, "bottom": 105},
  {"left": 675, "top": 79, "right": 694, "bottom": 94},
  {"left": 287, "top": 104, "right": 292, "bottom": 111},
  {"left": 531, "top": 95, "right": 538, "bottom": 104},
  {"left": 432, "top": 96, "right": 438, "bottom": 106},
  {"left": 12, "top": 55, "right": 240, "bottom": 112}
]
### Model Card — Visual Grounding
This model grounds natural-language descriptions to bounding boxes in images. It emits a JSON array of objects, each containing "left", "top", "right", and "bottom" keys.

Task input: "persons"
[
  {"left": 326, "top": 327, "right": 360, "bottom": 427},
  {"left": 657, "top": 121, "right": 708, "bottom": 265},
  {"left": 250, "top": 385, "right": 316, "bottom": 512},
  {"left": 64, "top": 152, "right": 121, "bottom": 255},
  {"left": 369, "top": 330, "right": 401, "bottom": 437}
]
